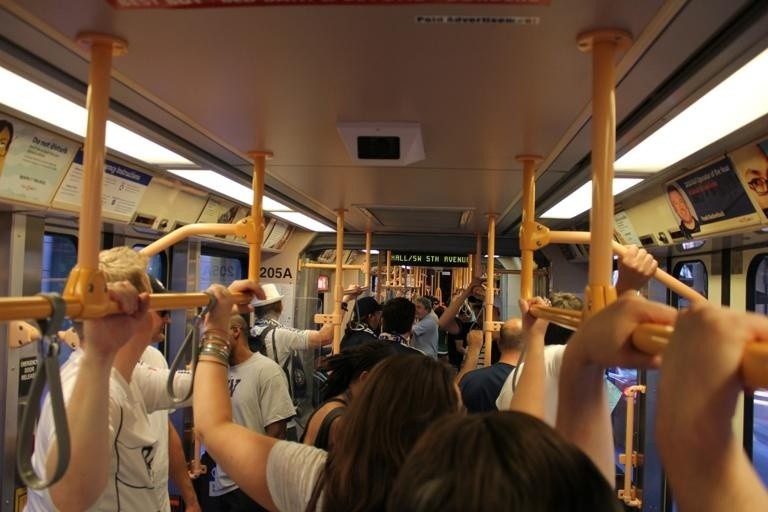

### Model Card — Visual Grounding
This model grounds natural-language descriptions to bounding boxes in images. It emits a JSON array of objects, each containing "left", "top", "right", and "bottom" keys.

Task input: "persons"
[
  {"left": 0, "top": 120, "right": 13, "bottom": 171},
  {"left": 1, "top": 246, "right": 768, "bottom": 512},
  {"left": 738, "top": 144, "right": 768, "bottom": 220},
  {"left": 666, "top": 184, "right": 699, "bottom": 238}
]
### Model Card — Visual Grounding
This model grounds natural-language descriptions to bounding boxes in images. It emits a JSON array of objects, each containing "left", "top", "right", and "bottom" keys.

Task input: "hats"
[
  {"left": 251, "top": 283, "right": 284, "bottom": 307},
  {"left": 353, "top": 298, "right": 386, "bottom": 317}
]
